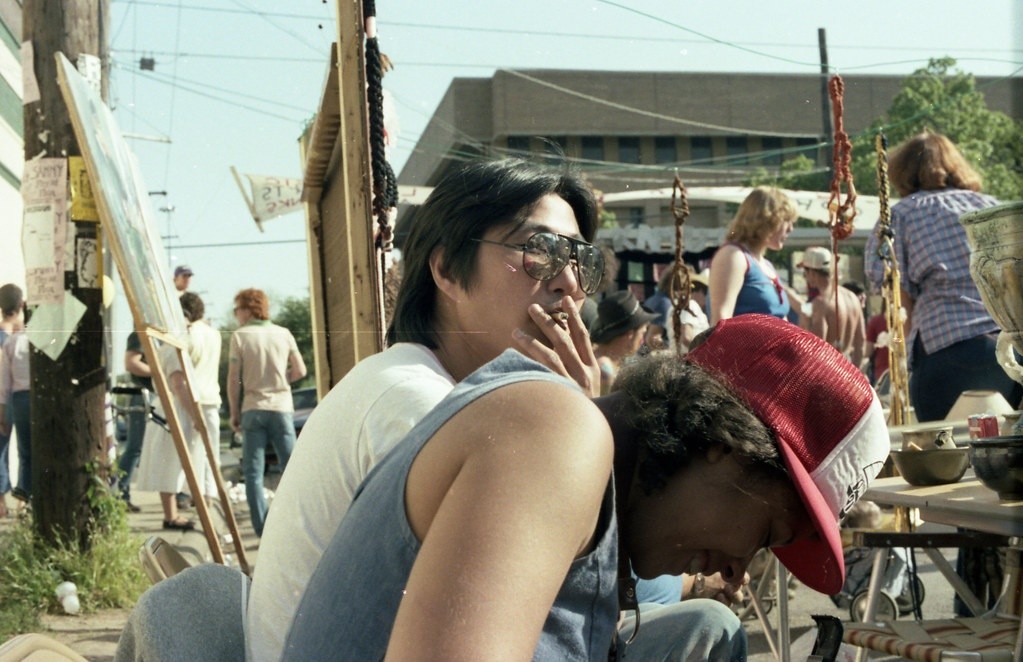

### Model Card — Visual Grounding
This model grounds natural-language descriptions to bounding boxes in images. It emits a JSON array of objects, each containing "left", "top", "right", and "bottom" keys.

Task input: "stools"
[{"left": 843, "top": 539, "right": 1023, "bottom": 662}]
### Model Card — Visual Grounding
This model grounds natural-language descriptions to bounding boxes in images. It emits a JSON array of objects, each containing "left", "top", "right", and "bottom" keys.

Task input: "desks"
[{"left": 851, "top": 474, "right": 1023, "bottom": 662}]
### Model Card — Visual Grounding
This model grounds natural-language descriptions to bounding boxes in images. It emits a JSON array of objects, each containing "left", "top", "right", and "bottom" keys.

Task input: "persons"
[
  {"left": 280, "top": 311, "right": 890, "bottom": 662},
  {"left": 248, "top": 157, "right": 604, "bottom": 662},
  {"left": 797, "top": 246, "right": 890, "bottom": 381},
  {"left": 180, "top": 293, "right": 222, "bottom": 508},
  {"left": 173, "top": 265, "right": 195, "bottom": 291},
  {"left": 0, "top": 283, "right": 31, "bottom": 518},
  {"left": 136, "top": 344, "right": 195, "bottom": 532},
  {"left": 706, "top": 186, "right": 799, "bottom": 328},
  {"left": 589, "top": 289, "right": 662, "bottom": 395},
  {"left": 865, "top": 133, "right": 1023, "bottom": 617},
  {"left": 108, "top": 331, "right": 191, "bottom": 512},
  {"left": 226, "top": 288, "right": 307, "bottom": 536},
  {"left": 639, "top": 260, "right": 710, "bottom": 357},
  {"left": 619, "top": 570, "right": 750, "bottom": 662}
]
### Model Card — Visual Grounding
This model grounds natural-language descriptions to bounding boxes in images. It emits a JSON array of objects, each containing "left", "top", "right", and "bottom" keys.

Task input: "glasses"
[
  {"left": 472, "top": 233, "right": 606, "bottom": 296},
  {"left": 234, "top": 306, "right": 244, "bottom": 314}
]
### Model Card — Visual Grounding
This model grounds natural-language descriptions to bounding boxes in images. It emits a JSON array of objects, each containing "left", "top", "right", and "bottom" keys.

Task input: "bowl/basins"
[
  {"left": 969, "top": 437, "right": 1023, "bottom": 500},
  {"left": 889, "top": 447, "right": 970, "bottom": 486}
]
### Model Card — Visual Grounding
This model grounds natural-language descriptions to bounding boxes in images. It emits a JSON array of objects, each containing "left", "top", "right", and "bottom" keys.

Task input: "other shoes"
[{"left": 163, "top": 517, "right": 195, "bottom": 530}]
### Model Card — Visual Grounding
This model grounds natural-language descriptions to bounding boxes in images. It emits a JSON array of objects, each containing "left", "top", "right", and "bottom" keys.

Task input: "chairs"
[
  {"left": 114, "top": 563, "right": 251, "bottom": 662},
  {"left": 138, "top": 536, "right": 191, "bottom": 584},
  {"left": 0, "top": 634, "right": 87, "bottom": 662}
]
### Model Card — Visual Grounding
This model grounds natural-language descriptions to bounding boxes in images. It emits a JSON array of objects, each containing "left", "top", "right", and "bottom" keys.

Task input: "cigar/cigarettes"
[{"left": 550, "top": 312, "right": 569, "bottom": 320}]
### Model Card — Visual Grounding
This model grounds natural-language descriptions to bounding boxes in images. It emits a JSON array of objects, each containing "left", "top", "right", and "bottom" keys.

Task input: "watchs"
[{"left": 693, "top": 573, "right": 705, "bottom": 597}]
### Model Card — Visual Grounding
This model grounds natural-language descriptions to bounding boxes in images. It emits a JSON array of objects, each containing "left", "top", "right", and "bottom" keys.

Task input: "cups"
[{"left": 55, "top": 581, "right": 80, "bottom": 614}]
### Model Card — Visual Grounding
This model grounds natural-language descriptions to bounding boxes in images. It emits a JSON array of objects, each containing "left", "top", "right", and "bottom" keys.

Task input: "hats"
[
  {"left": 589, "top": 289, "right": 661, "bottom": 346},
  {"left": 684, "top": 314, "right": 889, "bottom": 595},
  {"left": 690, "top": 267, "right": 711, "bottom": 287},
  {"left": 175, "top": 266, "right": 193, "bottom": 277},
  {"left": 797, "top": 247, "right": 831, "bottom": 272}
]
